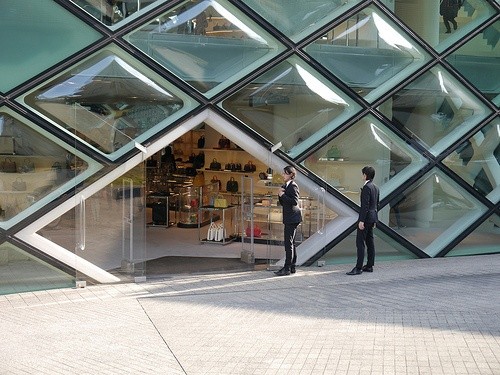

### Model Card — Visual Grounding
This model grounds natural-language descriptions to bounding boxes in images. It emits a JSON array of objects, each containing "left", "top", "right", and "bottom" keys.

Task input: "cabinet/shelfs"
[{"left": 0, "top": 147, "right": 360, "bottom": 246}]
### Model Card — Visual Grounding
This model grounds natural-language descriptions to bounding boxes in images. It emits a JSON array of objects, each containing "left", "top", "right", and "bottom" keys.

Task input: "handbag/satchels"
[
  {"left": 211, "top": 175, "right": 222, "bottom": 191},
  {"left": 246, "top": 226, "right": 262, "bottom": 237},
  {"left": 227, "top": 176, "right": 239, "bottom": 192},
  {"left": 190, "top": 214, "right": 198, "bottom": 224},
  {"left": 175, "top": 157, "right": 182, "bottom": 162},
  {"left": 244, "top": 161, "right": 257, "bottom": 172},
  {"left": 147, "top": 160, "right": 157, "bottom": 167},
  {"left": 214, "top": 195, "right": 229, "bottom": 208},
  {"left": 218, "top": 135, "right": 231, "bottom": 149},
  {"left": 207, "top": 222, "right": 227, "bottom": 241},
  {"left": 224, "top": 161, "right": 242, "bottom": 170},
  {"left": 191, "top": 199, "right": 198, "bottom": 206},
  {"left": 210, "top": 158, "right": 221, "bottom": 170},
  {"left": 198, "top": 136, "right": 205, "bottom": 148},
  {"left": 175, "top": 149, "right": 183, "bottom": 155},
  {"left": 192, "top": 173, "right": 205, "bottom": 186},
  {"left": 185, "top": 167, "right": 198, "bottom": 177},
  {"left": 188, "top": 151, "right": 205, "bottom": 168}
]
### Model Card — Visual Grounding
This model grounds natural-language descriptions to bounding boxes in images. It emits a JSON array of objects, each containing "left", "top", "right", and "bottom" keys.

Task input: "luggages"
[{"left": 152, "top": 200, "right": 169, "bottom": 225}]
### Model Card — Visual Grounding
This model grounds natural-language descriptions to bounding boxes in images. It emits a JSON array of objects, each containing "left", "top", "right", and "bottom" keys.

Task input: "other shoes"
[{"left": 276, "top": 268, "right": 296, "bottom": 276}]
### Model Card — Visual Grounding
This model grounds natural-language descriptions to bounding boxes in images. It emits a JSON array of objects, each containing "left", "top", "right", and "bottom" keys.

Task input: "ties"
[{"left": 283, "top": 184, "right": 287, "bottom": 190}]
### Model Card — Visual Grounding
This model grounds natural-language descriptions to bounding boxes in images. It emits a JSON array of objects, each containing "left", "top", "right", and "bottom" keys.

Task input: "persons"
[
  {"left": 346, "top": 166, "right": 380, "bottom": 275},
  {"left": 439, "top": 0, "right": 459, "bottom": 33},
  {"left": 390, "top": 167, "right": 405, "bottom": 226},
  {"left": 273, "top": 165, "right": 301, "bottom": 276}
]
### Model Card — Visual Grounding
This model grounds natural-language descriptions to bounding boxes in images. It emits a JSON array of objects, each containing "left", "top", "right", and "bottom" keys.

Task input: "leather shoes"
[
  {"left": 363, "top": 265, "right": 373, "bottom": 272},
  {"left": 346, "top": 267, "right": 363, "bottom": 275}
]
